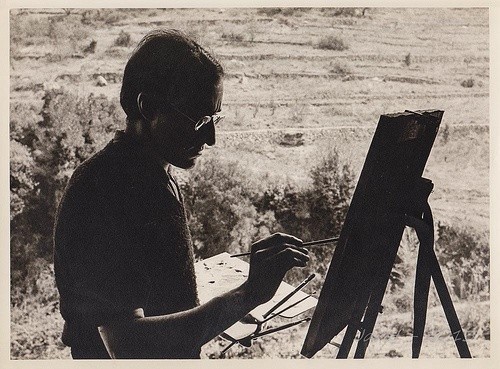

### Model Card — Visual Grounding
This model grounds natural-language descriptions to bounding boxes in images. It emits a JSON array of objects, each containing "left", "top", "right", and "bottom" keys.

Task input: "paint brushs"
[
  {"left": 249, "top": 318, "right": 312, "bottom": 342},
  {"left": 262, "top": 273, "right": 316, "bottom": 318},
  {"left": 219, "top": 291, "right": 318, "bottom": 354},
  {"left": 229, "top": 236, "right": 340, "bottom": 259}
]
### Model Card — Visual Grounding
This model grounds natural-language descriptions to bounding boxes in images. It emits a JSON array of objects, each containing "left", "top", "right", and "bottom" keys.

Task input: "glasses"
[{"left": 163, "top": 98, "right": 225, "bottom": 131}]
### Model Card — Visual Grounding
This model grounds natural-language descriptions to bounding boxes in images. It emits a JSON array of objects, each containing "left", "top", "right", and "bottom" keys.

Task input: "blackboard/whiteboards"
[{"left": 299, "top": 107, "right": 445, "bottom": 358}]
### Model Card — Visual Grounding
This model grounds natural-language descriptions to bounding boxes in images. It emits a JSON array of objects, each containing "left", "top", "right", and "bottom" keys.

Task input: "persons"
[{"left": 52, "top": 30, "right": 311, "bottom": 360}]
